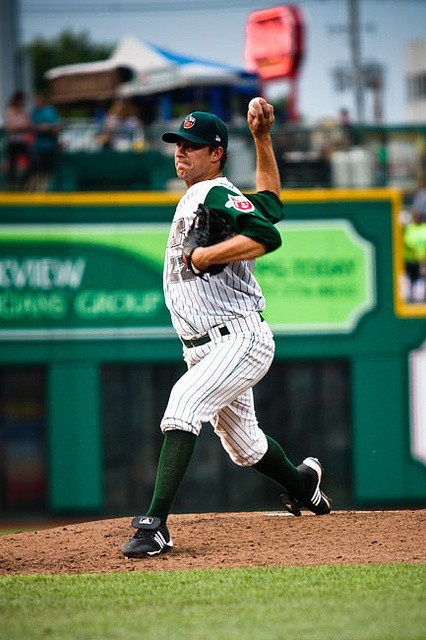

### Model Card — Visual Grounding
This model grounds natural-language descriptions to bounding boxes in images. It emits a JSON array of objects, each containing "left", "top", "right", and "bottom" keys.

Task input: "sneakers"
[
  {"left": 280, "top": 456, "right": 333, "bottom": 516},
  {"left": 121, "top": 515, "right": 174, "bottom": 558}
]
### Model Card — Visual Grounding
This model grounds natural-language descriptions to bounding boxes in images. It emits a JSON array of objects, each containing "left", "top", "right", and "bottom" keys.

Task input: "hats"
[{"left": 161, "top": 110, "right": 228, "bottom": 146}]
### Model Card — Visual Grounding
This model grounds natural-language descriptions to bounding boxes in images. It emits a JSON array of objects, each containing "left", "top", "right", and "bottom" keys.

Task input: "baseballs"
[{"left": 248, "top": 97, "right": 267, "bottom": 117}]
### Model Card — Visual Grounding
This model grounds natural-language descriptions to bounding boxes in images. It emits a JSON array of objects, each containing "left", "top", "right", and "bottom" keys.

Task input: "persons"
[
  {"left": 122, "top": 96, "right": 335, "bottom": 559},
  {"left": 95, "top": 99, "right": 143, "bottom": 151},
  {"left": 4, "top": 90, "right": 30, "bottom": 178},
  {"left": 27, "top": 94, "right": 63, "bottom": 174},
  {"left": 400, "top": 208, "right": 425, "bottom": 279}
]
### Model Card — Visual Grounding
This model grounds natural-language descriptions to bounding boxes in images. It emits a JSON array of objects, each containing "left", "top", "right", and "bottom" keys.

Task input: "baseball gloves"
[{"left": 182, "top": 202, "right": 232, "bottom": 276}]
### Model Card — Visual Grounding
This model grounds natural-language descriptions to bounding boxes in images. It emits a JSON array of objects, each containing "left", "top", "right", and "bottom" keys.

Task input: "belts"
[{"left": 182, "top": 327, "right": 230, "bottom": 348}]
